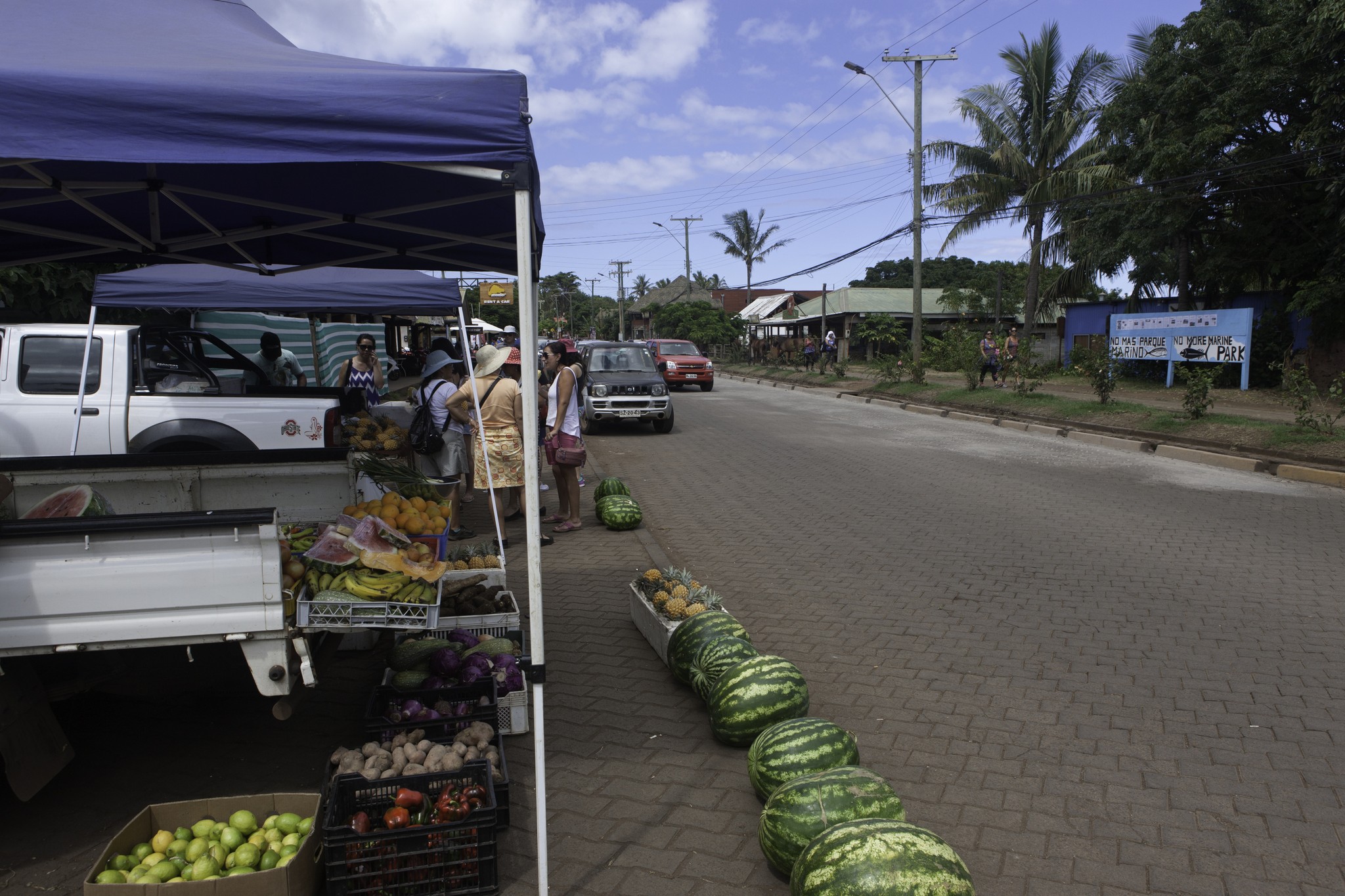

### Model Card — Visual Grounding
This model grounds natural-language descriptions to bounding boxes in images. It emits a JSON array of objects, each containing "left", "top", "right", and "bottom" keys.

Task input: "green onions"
[{"left": 344, "top": 450, "right": 444, "bottom": 492}]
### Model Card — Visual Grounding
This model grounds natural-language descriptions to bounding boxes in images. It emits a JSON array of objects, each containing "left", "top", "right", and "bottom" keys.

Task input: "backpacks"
[
  {"left": 820, "top": 339, "right": 826, "bottom": 353},
  {"left": 1002, "top": 349, "right": 1006, "bottom": 361},
  {"left": 407, "top": 380, "right": 451, "bottom": 456}
]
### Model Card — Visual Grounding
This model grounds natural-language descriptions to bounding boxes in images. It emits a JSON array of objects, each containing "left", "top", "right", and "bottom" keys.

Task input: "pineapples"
[
  {"left": 446, "top": 540, "right": 500, "bottom": 570},
  {"left": 461, "top": 401, "right": 468, "bottom": 412},
  {"left": 633, "top": 566, "right": 724, "bottom": 621},
  {"left": 341, "top": 412, "right": 409, "bottom": 450}
]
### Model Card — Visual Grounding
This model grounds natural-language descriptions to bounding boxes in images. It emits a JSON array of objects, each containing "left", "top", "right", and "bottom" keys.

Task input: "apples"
[
  {"left": 439, "top": 502, "right": 448, "bottom": 507},
  {"left": 280, "top": 540, "right": 305, "bottom": 590}
]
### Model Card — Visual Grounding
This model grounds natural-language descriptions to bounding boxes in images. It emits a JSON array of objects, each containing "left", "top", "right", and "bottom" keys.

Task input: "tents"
[{"left": 0, "top": 1, "right": 552, "bottom": 896}]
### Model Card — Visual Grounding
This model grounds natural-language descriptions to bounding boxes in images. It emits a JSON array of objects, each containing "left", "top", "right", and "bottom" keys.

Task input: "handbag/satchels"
[
  {"left": 539, "top": 407, "right": 548, "bottom": 421},
  {"left": 555, "top": 442, "right": 587, "bottom": 468}
]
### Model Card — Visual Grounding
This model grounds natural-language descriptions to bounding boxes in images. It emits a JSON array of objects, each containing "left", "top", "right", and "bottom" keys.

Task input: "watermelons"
[
  {"left": 18, "top": 485, "right": 116, "bottom": 518},
  {"left": 594, "top": 476, "right": 643, "bottom": 530},
  {"left": 300, "top": 514, "right": 412, "bottom": 574},
  {"left": 748, "top": 717, "right": 975, "bottom": 896},
  {"left": 666, "top": 611, "right": 810, "bottom": 746}
]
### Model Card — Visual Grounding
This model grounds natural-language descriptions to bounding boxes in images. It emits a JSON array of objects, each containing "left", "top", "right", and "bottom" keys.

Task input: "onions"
[{"left": 381, "top": 696, "right": 490, "bottom": 744}]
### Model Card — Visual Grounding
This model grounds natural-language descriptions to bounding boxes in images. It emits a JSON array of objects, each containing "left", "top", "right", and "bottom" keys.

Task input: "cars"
[
  {"left": 623, "top": 339, "right": 651, "bottom": 359},
  {"left": 537, "top": 336, "right": 559, "bottom": 366},
  {"left": 573, "top": 340, "right": 615, "bottom": 357}
]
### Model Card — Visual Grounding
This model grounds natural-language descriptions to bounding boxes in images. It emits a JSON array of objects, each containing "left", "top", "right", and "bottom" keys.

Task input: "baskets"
[{"left": 296, "top": 577, "right": 443, "bottom": 628}]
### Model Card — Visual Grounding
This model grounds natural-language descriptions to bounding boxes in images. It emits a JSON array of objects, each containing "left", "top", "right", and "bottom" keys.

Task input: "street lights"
[
  {"left": 843, "top": 60, "right": 924, "bottom": 383},
  {"left": 598, "top": 272, "right": 625, "bottom": 341}
]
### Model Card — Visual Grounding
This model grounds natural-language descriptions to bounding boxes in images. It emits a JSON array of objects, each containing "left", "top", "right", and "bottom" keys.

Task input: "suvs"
[
  {"left": 645, "top": 339, "right": 715, "bottom": 392},
  {"left": 576, "top": 341, "right": 674, "bottom": 435}
]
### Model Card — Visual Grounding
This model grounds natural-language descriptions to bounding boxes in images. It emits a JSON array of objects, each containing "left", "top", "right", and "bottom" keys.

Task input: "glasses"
[
  {"left": 429, "top": 348, "right": 436, "bottom": 350},
  {"left": 357, "top": 344, "right": 376, "bottom": 351},
  {"left": 986, "top": 333, "right": 993, "bottom": 335},
  {"left": 504, "top": 334, "right": 512, "bottom": 337},
  {"left": 451, "top": 364, "right": 453, "bottom": 367},
  {"left": 1011, "top": 330, "right": 1017, "bottom": 332},
  {"left": 542, "top": 352, "right": 557, "bottom": 359}
]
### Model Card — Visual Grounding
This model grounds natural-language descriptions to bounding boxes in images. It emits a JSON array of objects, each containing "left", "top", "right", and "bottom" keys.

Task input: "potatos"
[{"left": 330, "top": 721, "right": 502, "bottom": 801}]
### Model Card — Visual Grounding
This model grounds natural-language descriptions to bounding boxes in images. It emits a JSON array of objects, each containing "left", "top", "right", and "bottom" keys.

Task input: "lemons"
[{"left": 95, "top": 809, "right": 315, "bottom": 884}]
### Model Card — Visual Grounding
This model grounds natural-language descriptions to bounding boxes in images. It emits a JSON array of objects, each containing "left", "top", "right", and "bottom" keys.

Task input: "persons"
[
  {"left": 1003, "top": 327, "right": 1023, "bottom": 387},
  {"left": 979, "top": 330, "right": 1006, "bottom": 388},
  {"left": 245, "top": 324, "right": 589, "bottom": 550},
  {"left": 804, "top": 331, "right": 838, "bottom": 373}
]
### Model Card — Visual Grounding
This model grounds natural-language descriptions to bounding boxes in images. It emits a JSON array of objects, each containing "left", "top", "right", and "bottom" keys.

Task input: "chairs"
[
  {"left": 616, "top": 355, "right": 632, "bottom": 364},
  {"left": 592, "top": 355, "right": 614, "bottom": 368},
  {"left": 626, "top": 356, "right": 641, "bottom": 366}
]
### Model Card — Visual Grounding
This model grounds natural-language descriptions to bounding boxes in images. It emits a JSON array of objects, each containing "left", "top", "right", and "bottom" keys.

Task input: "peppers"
[
  {"left": 345, "top": 783, "right": 485, "bottom": 893},
  {"left": 282, "top": 520, "right": 319, "bottom": 554}
]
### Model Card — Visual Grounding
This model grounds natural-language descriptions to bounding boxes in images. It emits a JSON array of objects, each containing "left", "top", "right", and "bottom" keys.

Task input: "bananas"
[{"left": 306, "top": 569, "right": 438, "bottom": 610}]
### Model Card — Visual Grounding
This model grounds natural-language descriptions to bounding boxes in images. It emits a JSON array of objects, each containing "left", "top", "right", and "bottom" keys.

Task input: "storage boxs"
[
  {"left": 296, "top": 578, "right": 442, "bottom": 630},
  {"left": 498, "top": 670, "right": 529, "bottom": 736},
  {"left": 370, "top": 401, "right": 419, "bottom": 431},
  {"left": 442, "top": 555, "right": 507, "bottom": 590},
  {"left": 314, "top": 758, "right": 499, "bottom": 896},
  {"left": 394, "top": 591, "right": 520, "bottom": 647},
  {"left": 83, "top": 793, "right": 327, "bottom": 896},
  {"left": 321, "top": 733, "right": 510, "bottom": 831},
  {"left": 629, "top": 581, "right": 728, "bottom": 669},
  {"left": 406, "top": 518, "right": 450, "bottom": 561},
  {"left": 363, "top": 676, "right": 498, "bottom": 744}
]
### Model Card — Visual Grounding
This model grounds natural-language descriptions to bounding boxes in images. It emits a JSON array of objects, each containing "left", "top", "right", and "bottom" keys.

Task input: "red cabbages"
[
  {"left": 447, "top": 628, "right": 481, "bottom": 649},
  {"left": 421, "top": 647, "right": 523, "bottom": 698}
]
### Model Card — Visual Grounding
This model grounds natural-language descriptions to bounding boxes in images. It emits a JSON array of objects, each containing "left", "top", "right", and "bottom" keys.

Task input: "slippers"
[{"left": 461, "top": 496, "right": 475, "bottom": 502}]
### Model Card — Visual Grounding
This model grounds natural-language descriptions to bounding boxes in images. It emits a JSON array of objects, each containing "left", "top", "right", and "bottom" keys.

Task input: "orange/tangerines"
[{"left": 343, "top": 491, "right": 450, "bottom": 536}]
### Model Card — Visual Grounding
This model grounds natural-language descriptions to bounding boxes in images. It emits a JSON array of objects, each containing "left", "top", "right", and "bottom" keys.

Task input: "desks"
[{"left": 395, "top": 358, "right": 406, "bottom": 376}]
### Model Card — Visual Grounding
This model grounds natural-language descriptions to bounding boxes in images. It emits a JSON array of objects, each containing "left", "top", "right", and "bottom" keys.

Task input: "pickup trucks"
[{"left": 0, "top": 324, "right": 342, "bottom": 462}]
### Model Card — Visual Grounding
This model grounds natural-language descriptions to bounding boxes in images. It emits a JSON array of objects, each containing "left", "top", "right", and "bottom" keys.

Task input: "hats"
[
  {"left": 504, "top": 347, "right": 521, "bottom": 364},
  {"left": 260, "top": 331, "right": 282, "bottom": 358},
  {"left": 418, "top": 350, "right": 464, "bottom": 378},
  {"left": 557, "top": 338, "right": 578, "bottom": 352},
  {"left": 499, "top": 325, "right": 520, "bottom": 338},
  {"left": 470, "top": 344, "right": 512, "bottom": 378},
  {"left": 514, "top": 338, "right": 520, "bottom": 347}
]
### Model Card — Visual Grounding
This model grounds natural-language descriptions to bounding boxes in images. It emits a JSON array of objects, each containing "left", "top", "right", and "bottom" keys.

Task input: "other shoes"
[
  {"left": 1001, "top": 382, "right": 1008, "bottom": 387},
  {"left": 459, "top": 504, "right": 464, "bottom": 513},
  {"left": 580, "top": 473, "right": 585, "bottom": 482},
  {"left": 504, "top": 506, "right": 520, "bottom": 521},
  {"left": 493, "top": 537, "right": 509, "bottom": 549},
  {"left": 552, "top": 521, "right": 581, "bottom": 532},
  {"left": 520, "top": 506, "right": 546, "bottom": 516},
  {"left": 540, "top": 532, "right": 554, "bottom": 547},
  {"left": 823, "top": 364, "right": 826, "bottom": 368},
  {"left": 806, "top": 367, "right": 810, "bottom": 372},
  {"left": 811, "top": 366, "right": 815, "bottom": 371},
  {"left": 1017, "top": 383, "right": 1021, "bottom": 385},
  {"left": 578, "top": 477, "right": 585, "bottom": 487},
  {"left": 979, "top": 383, "right": 985, "bottom": 387},
  {"left": 448, "top": 525, "right": 477, "bottom": 540},
  {"left": 830, "top": 370, "right": 834, "bottom": 371},
  {"left": 541, "top": 514, "right": 570, "bottom": 523},
  {"left": 994, "top": 383, "right": 1004, "bottom": 388},
  {"left": 540, "top": 481, "right": 549, "bottom": 493}
]
